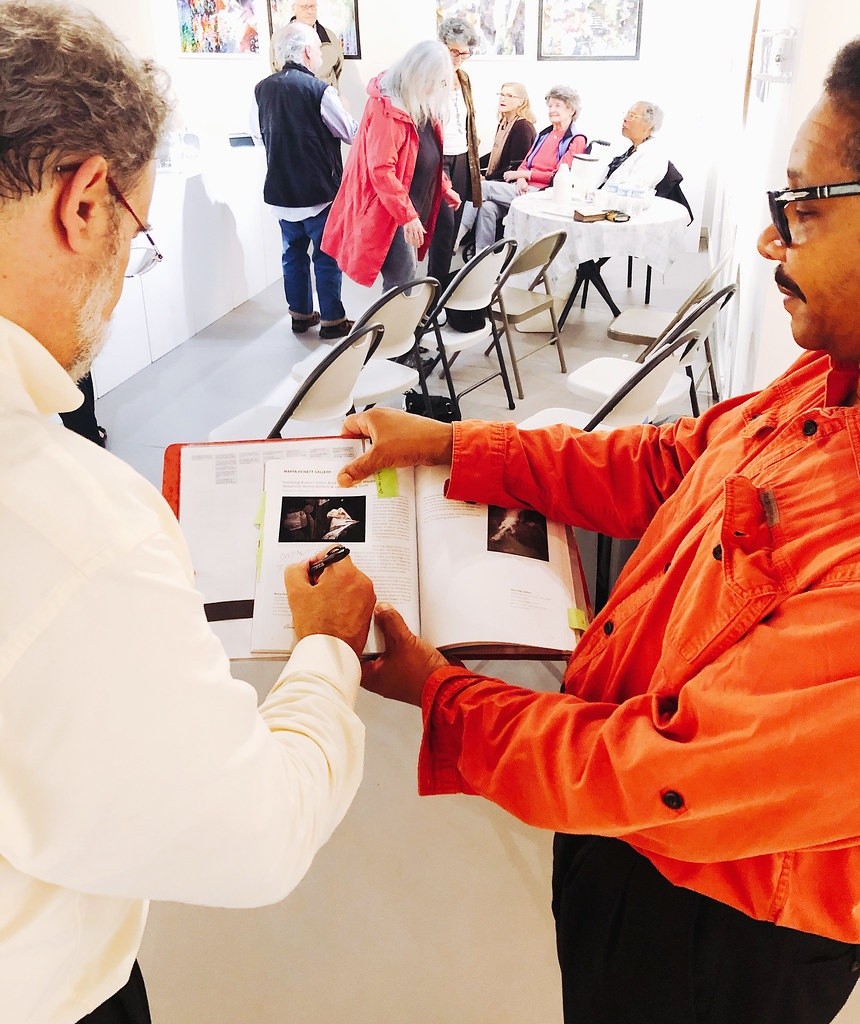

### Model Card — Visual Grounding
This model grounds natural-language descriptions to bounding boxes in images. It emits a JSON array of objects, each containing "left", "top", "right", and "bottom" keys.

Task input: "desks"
[{"left": 503, "top": 189, "right": 691, "bottom": 345}]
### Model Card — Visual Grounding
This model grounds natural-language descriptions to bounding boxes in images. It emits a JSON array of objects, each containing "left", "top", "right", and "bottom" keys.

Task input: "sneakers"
[
  {"left": 319, "top": 319, "right": 355, "bottom": 338},
  {"left": 292, "top": 311, "right": 321, "bottom": 332}
]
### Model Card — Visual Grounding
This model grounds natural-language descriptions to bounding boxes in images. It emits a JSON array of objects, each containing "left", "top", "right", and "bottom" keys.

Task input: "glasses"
[
  {"left": 297, "top": 3, "right": 319, "bottom": 12},
  {"left": 444, "top": 42, "right": 472, "bottom": 59},
  {"left": 496, "top": 93, "right": 520, "bottom": 101},
  {"left": 624, "top": 111, "right": 645, "bottom": 122},
  {"left": 766, "top": 181, "right": 860, "bottom": 249},
  {"left": 54, "top": 160, "right": 164, "bottom": 280}
]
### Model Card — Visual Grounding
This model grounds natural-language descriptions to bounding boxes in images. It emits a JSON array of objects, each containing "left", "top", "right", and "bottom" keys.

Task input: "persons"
[
  {"left": 419, "top": 18, "right": 482, "bottom": 333},
  {"left": 254, "top": 21, "right": 356, "bottom": 338},
  {"left": 514, "top": 101, "right": 668, "bottom": 333},
  {"left": 452, "top": 86, "right": 587, "bottom": 257},
  {"left": 337, "top": 38, "right": 860, "bottom": 1024},
  {"left": 269, "top": 1, "right": 344, "bottom": 95},
  {"left": 479, "top": 82, "right": 537, "bottom": 182},
  {"left": 319, "top": 38, "right": 455, "bottom": 369},
  {"left": 1, "top": 2, "right": 376, "bottom": 1024}
]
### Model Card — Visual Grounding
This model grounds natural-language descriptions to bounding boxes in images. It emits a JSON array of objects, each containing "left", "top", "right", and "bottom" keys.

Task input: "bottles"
[
  {"left": 553, "top": 163, "right": 572, "bottom": 211},
  {"left": 606, "top": 173, "right": 645, "bottom": 215}
]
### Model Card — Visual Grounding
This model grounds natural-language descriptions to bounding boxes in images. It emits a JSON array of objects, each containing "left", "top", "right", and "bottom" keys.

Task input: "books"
[{"left": 252, "top": 455, "right": 577, "bottom": 656}]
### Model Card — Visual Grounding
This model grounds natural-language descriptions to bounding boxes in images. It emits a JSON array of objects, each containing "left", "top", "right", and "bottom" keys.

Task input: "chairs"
[{"left": 210, "top": 233, "right": 736, "bottom": 616}]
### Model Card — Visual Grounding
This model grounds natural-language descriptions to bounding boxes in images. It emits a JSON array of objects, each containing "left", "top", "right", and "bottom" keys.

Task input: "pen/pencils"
[{"left": 310, "top": 546, "right": 350, "bottom": 574}]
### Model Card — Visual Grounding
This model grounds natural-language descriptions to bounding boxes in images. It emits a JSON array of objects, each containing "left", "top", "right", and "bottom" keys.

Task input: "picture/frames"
[
  {"left": 174, "top": 0, "right": 265, "bottom": 58},
  {"left": 538, "top": 0, "right": 643, "bottom": 61},
  {"left": 429, "top": 0, "right": 532, "bottom": 62},
  {"left": 265, "top": 0, "right": 361, "bottom": 59}
]
